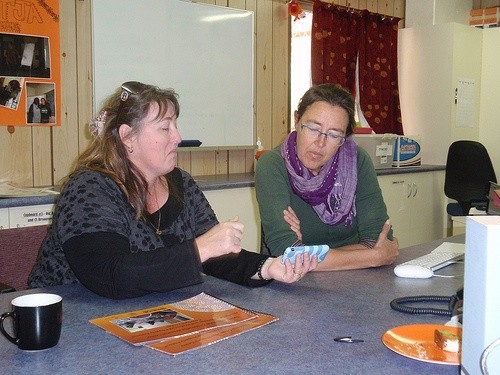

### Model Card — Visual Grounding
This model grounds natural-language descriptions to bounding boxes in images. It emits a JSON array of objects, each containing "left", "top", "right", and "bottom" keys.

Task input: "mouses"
[{"left": 393, "top": 265, "right": 434, "bottom": 279}]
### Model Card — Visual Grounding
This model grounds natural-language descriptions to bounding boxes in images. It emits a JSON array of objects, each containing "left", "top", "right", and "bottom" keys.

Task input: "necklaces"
[{"left": 133, "top": 189, "right": 162, "bottom": 235}]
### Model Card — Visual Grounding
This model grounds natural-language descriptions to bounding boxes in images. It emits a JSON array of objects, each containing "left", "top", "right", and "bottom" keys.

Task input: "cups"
[{"left": 0, "top": 292, "right": 62, "bottom": 353}]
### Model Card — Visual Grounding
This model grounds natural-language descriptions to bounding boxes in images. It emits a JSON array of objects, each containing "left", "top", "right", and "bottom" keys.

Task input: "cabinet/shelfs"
[{"left": 375, "top": 164, "right": 448, "bottom": 249}]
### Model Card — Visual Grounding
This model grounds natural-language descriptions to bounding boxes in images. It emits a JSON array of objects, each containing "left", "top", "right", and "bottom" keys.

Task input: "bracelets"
[{"left": 258, "top": 256, "right": 274, "bottom": 282}]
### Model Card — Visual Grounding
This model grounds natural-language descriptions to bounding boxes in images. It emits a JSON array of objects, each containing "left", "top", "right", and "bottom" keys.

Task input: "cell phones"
[{"left": 281, "top": 245, "right": 329, "bottom": 263}]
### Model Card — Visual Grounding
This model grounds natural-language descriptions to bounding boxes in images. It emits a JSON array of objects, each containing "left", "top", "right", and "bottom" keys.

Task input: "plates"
[{"left": 382, "top": 323, "right": 461, "bottom": 366}]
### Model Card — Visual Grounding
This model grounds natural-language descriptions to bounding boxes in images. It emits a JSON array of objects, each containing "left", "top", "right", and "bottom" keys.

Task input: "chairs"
[
  {"left": 1, "top": 224, "right": 52, "bottom": 294},
  {"left": 445, "top": 140, "right": 497, "bottom": 237}
]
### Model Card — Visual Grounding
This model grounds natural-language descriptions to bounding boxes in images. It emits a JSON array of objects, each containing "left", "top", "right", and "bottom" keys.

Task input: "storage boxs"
[{"left": 352, "top": 133, "right": 399, "bottom": 170}]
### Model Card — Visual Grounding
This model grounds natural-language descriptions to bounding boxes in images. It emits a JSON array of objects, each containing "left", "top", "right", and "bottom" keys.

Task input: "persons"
[
  {"left": 28, "top": 97, "right": 52, "bottom": 124},
  {"left": 27, "top": 80, "right": 318, "bottom": 300},
  {"left": 254, "top": 84, "right": 400, "bottom": 273},
  {"left": 0, "top": 79, "right": 21, "bottom": 109}
]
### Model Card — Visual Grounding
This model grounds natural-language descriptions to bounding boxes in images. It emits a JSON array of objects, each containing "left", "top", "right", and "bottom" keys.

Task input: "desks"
[{"left": 0, "top": 233, "right": 466, "bottom": 375}]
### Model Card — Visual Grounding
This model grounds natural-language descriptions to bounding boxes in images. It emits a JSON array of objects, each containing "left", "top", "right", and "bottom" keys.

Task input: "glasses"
[
  {"left": 116, "top": 80, "right": 147, "bottom": 128},
  {"left": 299, "top": 116, "right": 347, "bottom": 146}
]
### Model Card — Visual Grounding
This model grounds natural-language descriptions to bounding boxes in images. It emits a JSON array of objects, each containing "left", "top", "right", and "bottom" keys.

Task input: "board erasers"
[{"left": 178, "top": 140, "right": 202, "bottom": 147}]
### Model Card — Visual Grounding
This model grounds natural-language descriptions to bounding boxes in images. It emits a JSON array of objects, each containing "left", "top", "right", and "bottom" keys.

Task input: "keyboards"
[{"left": 398, "top": 249, "right": 465, "bottom": 272}]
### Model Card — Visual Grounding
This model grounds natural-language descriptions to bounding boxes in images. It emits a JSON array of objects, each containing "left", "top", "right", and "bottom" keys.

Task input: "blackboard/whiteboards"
[{"left": 89, "top": 0, "right": 258, "bottom": 152}]
